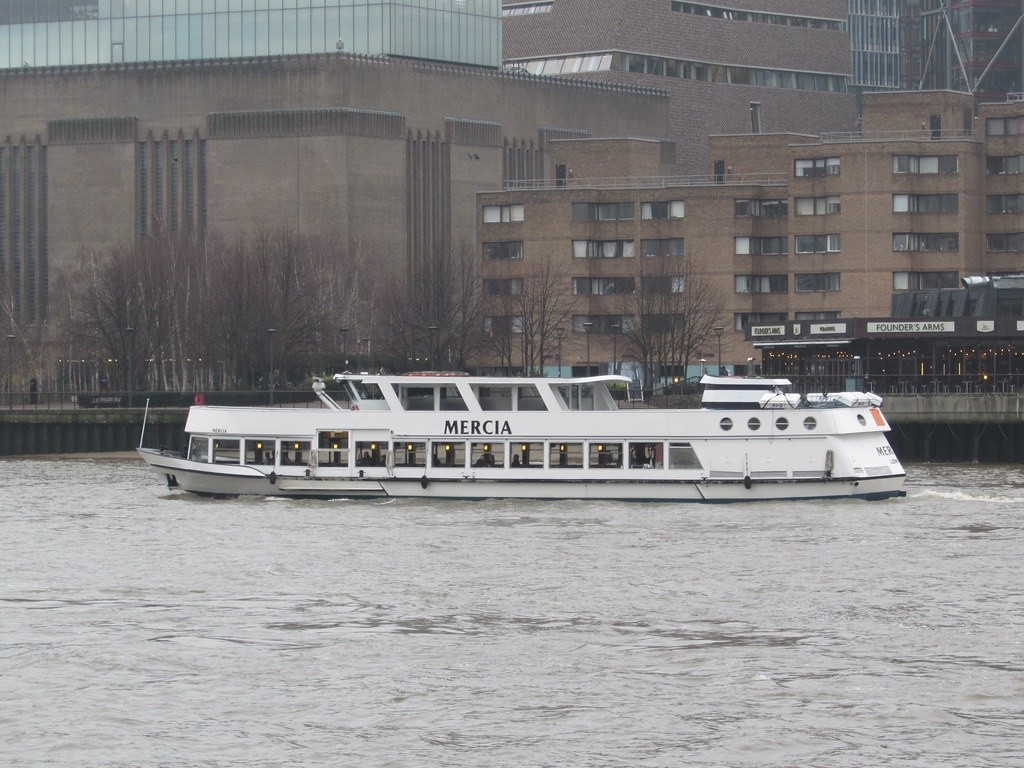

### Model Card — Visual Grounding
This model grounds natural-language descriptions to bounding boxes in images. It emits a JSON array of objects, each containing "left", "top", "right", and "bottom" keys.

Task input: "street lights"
[
  {"left": 713, "top": 326, "right": 725, "bottom": 377},
  {"left": 581, "top": 322, "right": 596, "bottom": 377},
  {"left": 339, "top": 326, "right": 350, "bottom": 373},
  {"left": 265, "top": 328, "right": 278, "bottom": 408},
  {"left": 554, "top": 327, "right": 567, "bottom": 377},
  {"left": 610, "top": 323, "right": 623, "bottom": 375}
]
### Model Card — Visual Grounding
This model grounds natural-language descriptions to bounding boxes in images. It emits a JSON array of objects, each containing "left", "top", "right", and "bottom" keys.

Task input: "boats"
[{"left": 132, "top": 370, "right": 910, "bottom": 503}]
